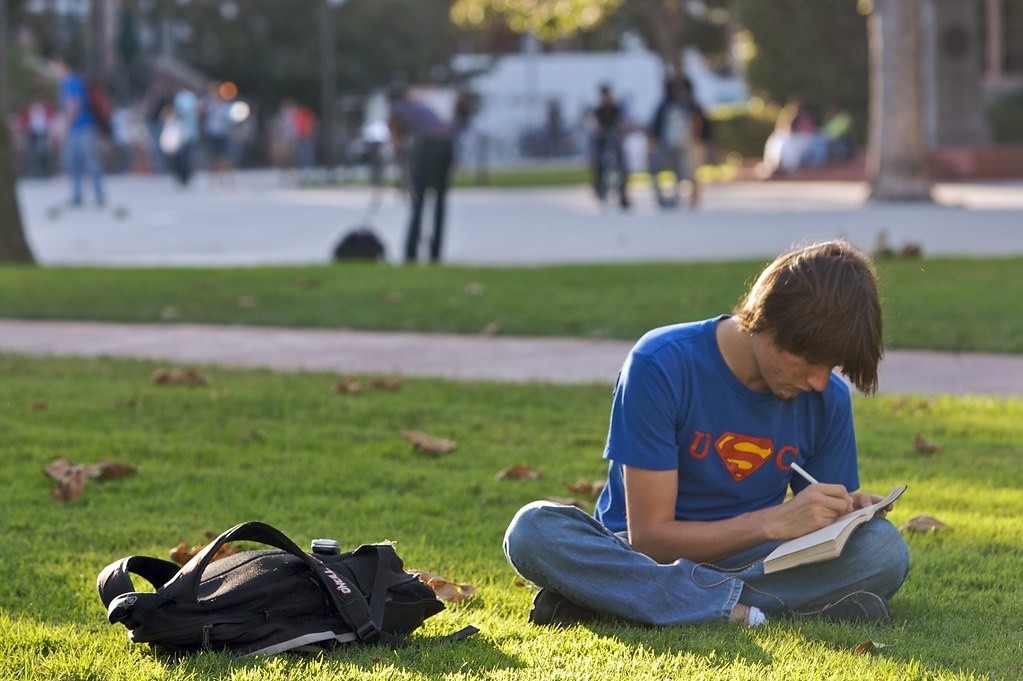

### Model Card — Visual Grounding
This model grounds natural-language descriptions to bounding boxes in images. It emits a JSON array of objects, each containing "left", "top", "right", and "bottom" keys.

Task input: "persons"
[
  {"left": 503, "top": 240, "right": 910, "bottom": 631},
  {"left": 158, "top": 83, "right": 233, "bottom": 188},
  {"left": 54, "top": 50, "right": 106, "bottom": 206},
  {"left": 644, "top": 79, "right": 709, "bottom": 210},
  {"left": 23, "top": 88, "right": 56, "bottom": 178},
  {"left": 362, "top": 81, "right": 455, "bottom": 264},
  {"left": 584, "top": 85, "right": 634, "bottom": 209},
  {"left": 756, "top": 91, "right": 853, "bottom": 180}
]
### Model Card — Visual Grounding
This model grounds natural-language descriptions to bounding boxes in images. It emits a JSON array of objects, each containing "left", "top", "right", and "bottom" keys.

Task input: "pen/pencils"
[{"left": 789, "top": 462, "right": 820, "bottom": 484}]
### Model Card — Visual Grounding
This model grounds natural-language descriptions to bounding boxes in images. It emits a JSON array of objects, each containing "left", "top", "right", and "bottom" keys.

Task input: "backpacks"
[{"left": 96, "top": 520, "right": 481, "bottom": 658}]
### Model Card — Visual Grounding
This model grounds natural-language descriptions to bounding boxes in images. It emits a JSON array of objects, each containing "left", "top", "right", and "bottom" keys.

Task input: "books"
[{"left": 762, "top": 485, "right": 907, "bottom": 577}]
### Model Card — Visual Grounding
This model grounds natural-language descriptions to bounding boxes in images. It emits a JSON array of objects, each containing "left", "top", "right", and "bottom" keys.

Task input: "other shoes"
[
  {"left": 760, "top": 587, "right": 890, "bottom": 629},
  {"left": 528, "top": 588, "right": 596, "bottom": 627}
]
